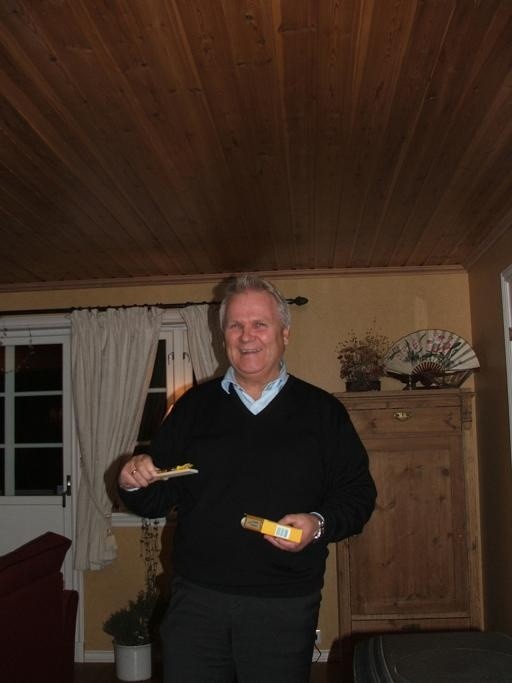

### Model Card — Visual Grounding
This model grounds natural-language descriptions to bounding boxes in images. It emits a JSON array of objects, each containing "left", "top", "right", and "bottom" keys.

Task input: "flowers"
[{"left": 333, "top": 317, "right": 395, "bottom": 384}]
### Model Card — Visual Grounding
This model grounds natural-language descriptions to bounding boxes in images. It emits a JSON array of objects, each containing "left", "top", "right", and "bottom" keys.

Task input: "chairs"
[{"left": 0, "top": 528, "right": 81, "bottom": 683}]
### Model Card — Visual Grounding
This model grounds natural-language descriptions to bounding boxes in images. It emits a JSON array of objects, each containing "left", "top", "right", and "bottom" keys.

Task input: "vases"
[{"left": 345, "top": 379, "right": 382, "bottom": 393}]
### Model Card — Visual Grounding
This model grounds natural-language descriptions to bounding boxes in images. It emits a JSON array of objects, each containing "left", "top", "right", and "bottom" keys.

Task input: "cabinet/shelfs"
[{"left": 330, "top": 387, "right": 484, "bottom": 642}]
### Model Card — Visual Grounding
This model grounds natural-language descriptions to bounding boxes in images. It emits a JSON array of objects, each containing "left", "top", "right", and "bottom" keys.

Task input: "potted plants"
[{"left": 100, "top": 603, "right": 156, "bottom": 681}]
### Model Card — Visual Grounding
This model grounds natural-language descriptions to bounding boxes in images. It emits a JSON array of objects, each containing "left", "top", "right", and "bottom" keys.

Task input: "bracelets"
[{"left": 314, "top": 521, "right": 323, "bottom": 541}]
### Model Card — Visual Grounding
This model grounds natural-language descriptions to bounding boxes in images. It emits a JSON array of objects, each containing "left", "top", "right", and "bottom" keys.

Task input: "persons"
[{"left": 117, "top": 276, "right": 377, "bottom": 683}]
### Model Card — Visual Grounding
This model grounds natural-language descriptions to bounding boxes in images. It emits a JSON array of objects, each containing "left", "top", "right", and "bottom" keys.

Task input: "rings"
[{"left": 130, "top": 469, "right": 136, "bottom": 474}]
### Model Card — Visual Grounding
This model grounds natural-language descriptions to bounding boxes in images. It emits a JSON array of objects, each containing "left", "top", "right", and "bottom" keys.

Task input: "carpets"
[{"left": 325, "top": 626, "right": 511, "bottom": 683}]
